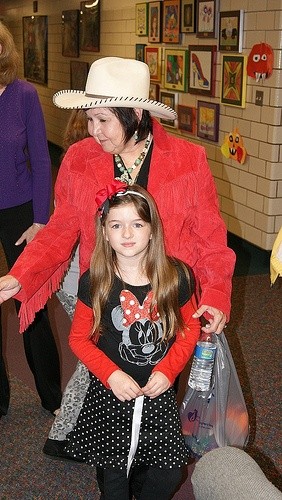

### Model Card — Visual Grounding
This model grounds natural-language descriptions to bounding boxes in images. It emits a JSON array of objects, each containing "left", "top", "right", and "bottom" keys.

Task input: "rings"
[{"left": 223, "top": 325, "right": 228, "bottom": 329}]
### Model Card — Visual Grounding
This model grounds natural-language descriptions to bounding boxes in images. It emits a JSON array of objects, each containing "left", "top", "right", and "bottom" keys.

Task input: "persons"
[
  {"left": 0, "top": 57, "right": 236, "bottom": 464},
  {"left": 0, "top": 21, "right": 63, "bottom": 418},
  {"left": 43, "top": 173, "right": 202, "bottom": 500}
]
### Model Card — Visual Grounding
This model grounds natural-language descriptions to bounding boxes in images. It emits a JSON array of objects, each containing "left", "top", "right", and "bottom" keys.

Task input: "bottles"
[{"left": 187, "top": 325, "right": 217, "bottom": 393}]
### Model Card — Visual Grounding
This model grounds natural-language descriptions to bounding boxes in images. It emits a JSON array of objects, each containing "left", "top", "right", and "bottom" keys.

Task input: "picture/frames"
[
  {"left": 177, "top": 104, "right": 197, "bottom": 135},
  {"left": 159, "top": 90, "right": 179, "bottom": 128},
  {"left": 197, "top": 100, "right": 218, "bottom": 142},
  {"left": 79, "top": 0, "right": 100, "bottom": 52},
  {"left": 217, "top": 10, "right": 243, "bottom": 53},
  {"left": 136, "top": 43, "right": 147, "bottom": 62},
  {"left": 70, "top": 60, "right": 89, "bottom": 91},
  {"left": 221, "top": 55, "right": 246, "bottom": 108},
  {"left": 144, "top": 46, "right": 162, "bottom": 82},
  {"left": 149, "top": 83, "right": 159, "bottom": 102},
  {"left": 164, "top": 48, "right": 188, "bottom": 93},
  {"left": 136, "top": 0, "right": 220, "bottom": 44},
  {"left": 62, "top": 10, "right": 79, "bottom": 57},
  {"left": 188, "top": 44, "right": 216, "bottom": 98},
  {"left": 23, "top": 15, "right": 48, "bottom": 85}
]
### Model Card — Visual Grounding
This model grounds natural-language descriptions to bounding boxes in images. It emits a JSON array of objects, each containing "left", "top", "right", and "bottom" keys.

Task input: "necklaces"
[{"left": 115, "top": 132, "right": 151, "bottom": 186}]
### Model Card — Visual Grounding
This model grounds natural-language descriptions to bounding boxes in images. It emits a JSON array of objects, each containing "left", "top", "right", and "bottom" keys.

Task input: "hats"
[{"left": 53, "top": 56, "right": 178, "bottom": 121}]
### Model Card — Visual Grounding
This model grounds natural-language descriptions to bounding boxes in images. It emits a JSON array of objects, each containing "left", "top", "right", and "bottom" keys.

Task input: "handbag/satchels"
[{"left": 179, "top": 327, "right": 250, "bottom": 459}]
